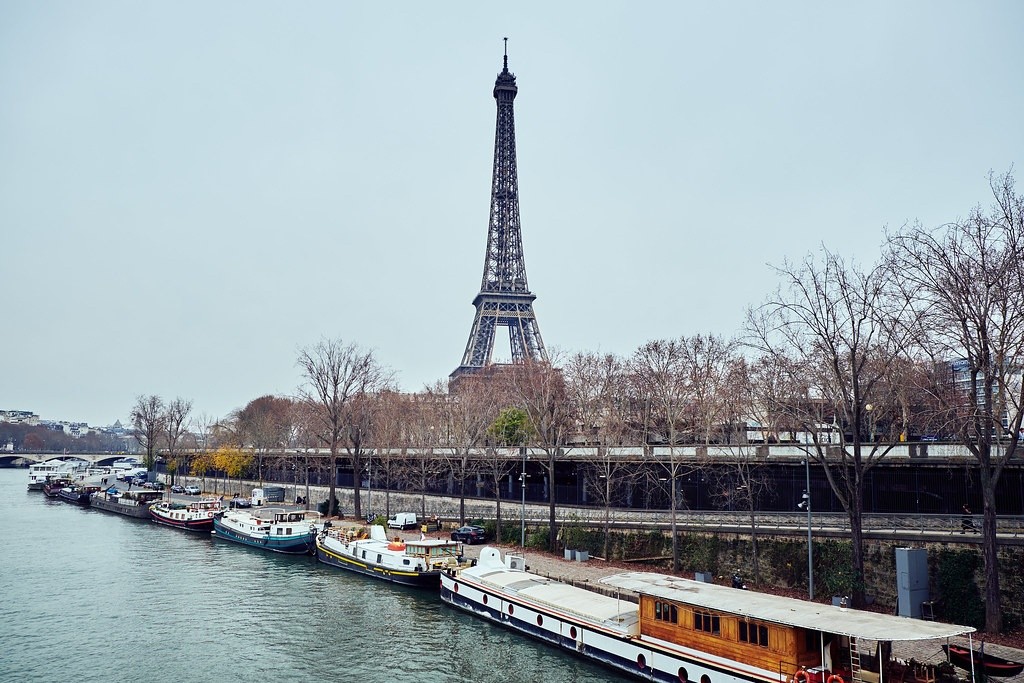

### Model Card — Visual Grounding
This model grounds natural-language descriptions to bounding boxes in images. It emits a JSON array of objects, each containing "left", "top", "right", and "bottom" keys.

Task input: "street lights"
[
  {"left": 798, "top": 501, "right": 814, "bottom": 600},
  {"left": 517, "top": 475, "right": 525, "bottom": 548}
]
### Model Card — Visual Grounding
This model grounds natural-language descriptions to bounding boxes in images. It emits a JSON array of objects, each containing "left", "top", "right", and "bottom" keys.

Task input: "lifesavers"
[
  {"left": 208, "top": 512, "right": 214, "bottom": 518},
  {"left": 793, "top": 670, "right": 811, "bottom": 683},
  {"left": 826, "top": 674, "right": 844, "bottom": 683}
]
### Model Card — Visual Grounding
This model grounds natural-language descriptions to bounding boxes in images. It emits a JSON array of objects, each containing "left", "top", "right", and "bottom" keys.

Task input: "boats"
[
  {"left": 70, "top": 461, "right": 149, "bottom": 485},
  {"left": 88, "top": 484, "right": 165, "bottom": 519},
  {"left": 436, "top": 548, "right": 992, "bottom": 683},
  {"left": 941, "top": 644, "right": 1024, "bottom": 677},
  {"left": 315, "top": 520, "right": 478, "bottom": 589},
  {"left": 147, "top": 498, "right": 228, "bottom": 532},
  {"left": 213, "top": 506, "right": 335, "bottom": 554},
  {"left": 57, "top": 482, "right": 101, "bottom": 505},
  {"left": 43, "top": 477, "right": 73, "bottom": 498},
  {"left": 27, "top": 460, "right": 69, "bottom": 490}
]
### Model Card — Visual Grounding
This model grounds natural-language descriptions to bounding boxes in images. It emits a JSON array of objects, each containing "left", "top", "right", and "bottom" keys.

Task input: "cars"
[
  {"left": 230, "top": 498, "right": 252, "bottom": 509},
  {"left": 450, "top": 524, "right": 486, "bottom": 546},
  {"left": 169, "top": 485, "right": 185, "bottom": 493},
  {"left": 133, "top": 478, "right": 163, "bottom": 491},
  {"left": 185, "top": 486, "right": 201, "bottom": 496}
]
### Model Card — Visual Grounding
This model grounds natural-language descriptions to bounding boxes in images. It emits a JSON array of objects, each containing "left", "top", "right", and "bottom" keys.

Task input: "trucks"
[{"left": 387, "top": 513, "right": 417, "bottom": 531}]
